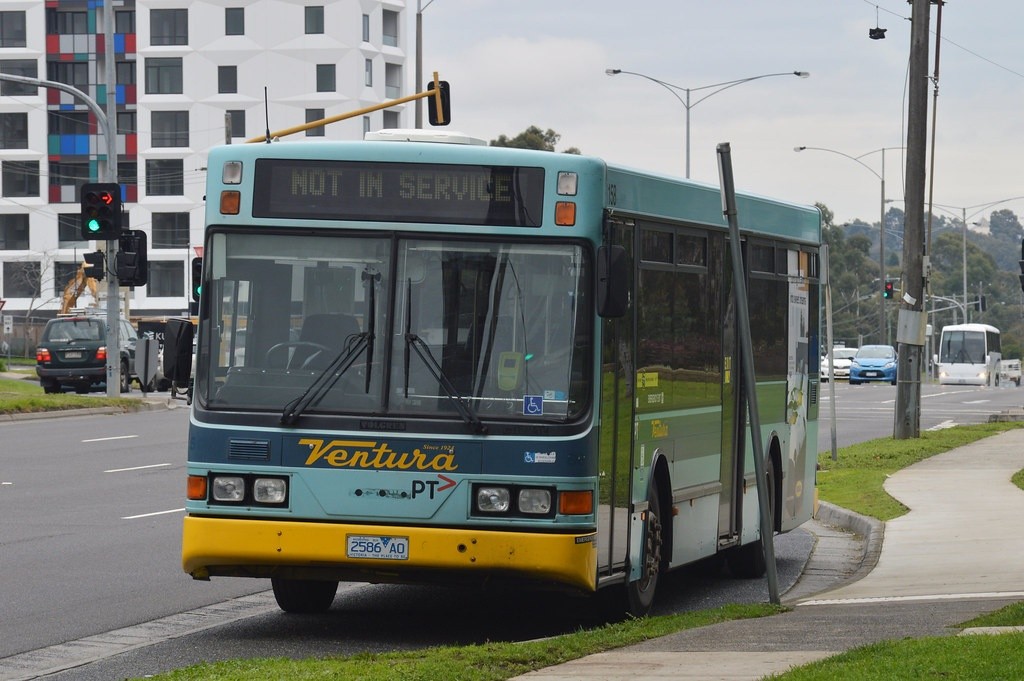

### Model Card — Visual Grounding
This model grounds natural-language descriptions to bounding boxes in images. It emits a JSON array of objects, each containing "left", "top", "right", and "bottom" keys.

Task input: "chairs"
[{"left": 284, "top": 313, "right": 366, "bottom": 373}]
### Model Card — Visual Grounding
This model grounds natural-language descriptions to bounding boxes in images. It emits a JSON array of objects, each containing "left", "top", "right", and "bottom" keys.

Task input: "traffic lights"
[
  {"left": 192, "top": 258, "right": 209, "bottom": 302},
  {"left": 84, "top": 250, "right": 105, "bottom": 280},
  {"left": 884, "top": 283, "right": 893, "bottom": 298},
  {"left": 81, "top": 182, "right": 122, "bottom": 241}
]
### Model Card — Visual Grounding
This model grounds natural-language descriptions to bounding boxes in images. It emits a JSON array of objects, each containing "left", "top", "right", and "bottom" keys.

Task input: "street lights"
[
  {"left": 606, "top": 68, "right": 809, "bottom": 177},
  {"left": 883, "top": 196, "right": 1023, "bottom": 324},
  {"left": 844, "top": 222, "right": 982, "bottom": 378},
  {"left": 793, "top": 146, "right": 886, "bottom": 343}
]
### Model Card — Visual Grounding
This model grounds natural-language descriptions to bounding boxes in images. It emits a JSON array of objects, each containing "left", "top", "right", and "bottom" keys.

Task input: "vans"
[{"left": 234, "top": 326, "right": 300, "bottom": 371}]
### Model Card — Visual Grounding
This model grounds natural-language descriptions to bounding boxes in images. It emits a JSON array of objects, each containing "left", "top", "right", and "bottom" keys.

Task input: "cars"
[
  {"left": 848, "top": 344, "right": 899, "bottom": 385},
  {"left": 821, "top": 348, "right": 857, "bottom": 383}
]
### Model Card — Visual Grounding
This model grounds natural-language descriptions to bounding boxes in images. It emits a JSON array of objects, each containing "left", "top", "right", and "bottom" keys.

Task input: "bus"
[
  {"left": 163, "top": 128, "right": 823, "bottom": 617},
  {"left": 933, "top": 323, "right": 1002, "bottom": 386}
]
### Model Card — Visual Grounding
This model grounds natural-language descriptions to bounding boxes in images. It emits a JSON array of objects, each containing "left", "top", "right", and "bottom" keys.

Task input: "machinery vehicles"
[{"left": 60, "top": 261, "right": 138, "bottom": 334}]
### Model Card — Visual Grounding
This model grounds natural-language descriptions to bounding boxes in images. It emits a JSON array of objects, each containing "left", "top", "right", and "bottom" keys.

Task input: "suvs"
[
  {"left": 35, "top": 313, "right": 140, "bottom": 392},
  {"left": 155, "top": 337, "right": 197, "bottom": 392}
]
